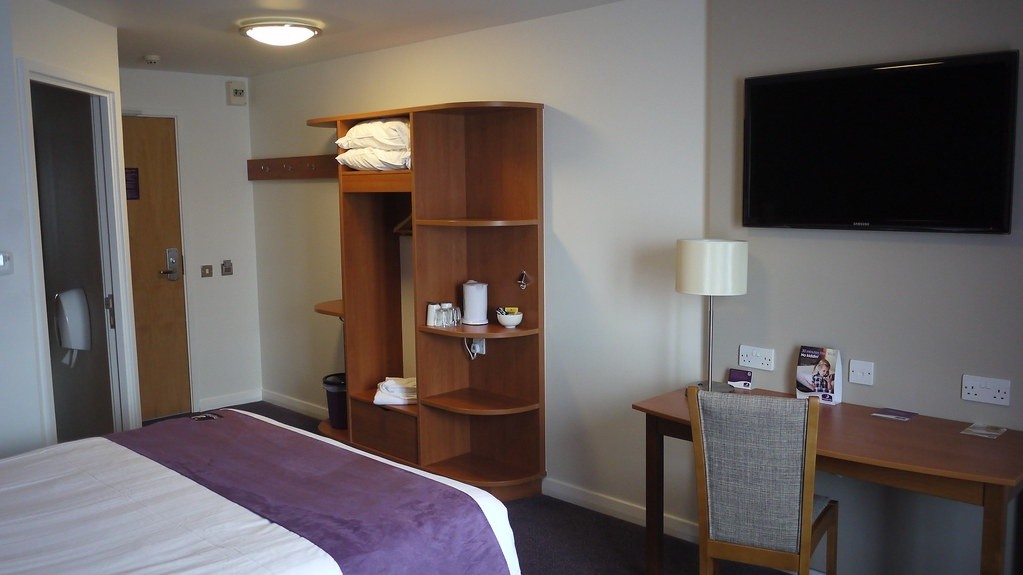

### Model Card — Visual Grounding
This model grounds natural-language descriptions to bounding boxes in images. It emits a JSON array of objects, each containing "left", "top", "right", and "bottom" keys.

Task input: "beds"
[{"left": 0, "top": 405, "right": 522, "bottom": 575}]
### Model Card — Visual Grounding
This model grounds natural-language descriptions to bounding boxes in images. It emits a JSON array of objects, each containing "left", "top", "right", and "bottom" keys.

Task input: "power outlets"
[
  {"left": 739, "top": 344, "right": 776, "bottom": 373},
  {"left": 961, "top": 373, "right": 1012, "bottom": 407}
]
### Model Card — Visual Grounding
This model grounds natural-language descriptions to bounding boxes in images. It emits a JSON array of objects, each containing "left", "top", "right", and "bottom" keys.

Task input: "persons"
[{"left": 811, "top": 358, "right": 833, "bottom": 394}]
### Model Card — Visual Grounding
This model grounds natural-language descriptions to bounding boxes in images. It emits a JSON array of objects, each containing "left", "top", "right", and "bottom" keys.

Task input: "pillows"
[{"left": 333, "top": 115, "right": 412, "bottom": 172}]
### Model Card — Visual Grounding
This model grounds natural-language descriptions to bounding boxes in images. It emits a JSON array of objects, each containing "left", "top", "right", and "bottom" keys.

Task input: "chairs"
[{"left": 685, "top": 386, "right": 841, "bottom": 575}]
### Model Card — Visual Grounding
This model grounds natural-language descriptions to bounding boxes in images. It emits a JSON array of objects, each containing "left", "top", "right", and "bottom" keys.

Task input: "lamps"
[
  {"left": 237, "top": 22, "right": 322, "bottom": 47},
  {"left": 674, "top": 237, "right": 749, "bottom": 398}
]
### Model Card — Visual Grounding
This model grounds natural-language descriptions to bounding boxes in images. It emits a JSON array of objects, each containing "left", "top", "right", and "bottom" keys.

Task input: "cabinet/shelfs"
[{"left": 305, "top": 101, "right": 548, "bottom": 503}]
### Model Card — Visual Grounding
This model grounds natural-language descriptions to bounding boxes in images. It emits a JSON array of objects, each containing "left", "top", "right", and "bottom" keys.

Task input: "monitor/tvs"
[{"left": 742, "top": 48, "right": 1020, "bottom": 235}]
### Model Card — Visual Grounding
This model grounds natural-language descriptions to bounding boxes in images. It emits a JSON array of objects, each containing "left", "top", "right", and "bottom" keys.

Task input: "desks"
[{"left": 631, "top": 380, "right": 1022, "bottom": 575}]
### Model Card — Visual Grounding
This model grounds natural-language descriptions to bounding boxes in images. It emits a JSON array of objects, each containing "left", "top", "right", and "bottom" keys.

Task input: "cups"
[
  {"left": 427, "top": 304, "right": 441, "bottom": 326},
  {"left": 448, "top": 307, "right": 461, "bottom": 325},
  {"left": 441, "top": 302, "right": 453, "bottom": 325},
  {"left": 435, "top": 308, "right": 450, "bottom": 328}
]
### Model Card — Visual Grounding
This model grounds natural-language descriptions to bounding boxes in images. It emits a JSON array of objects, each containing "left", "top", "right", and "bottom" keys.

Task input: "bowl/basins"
[{"left": 497, "top": 312, "right": 523, "bottom": 328}]
